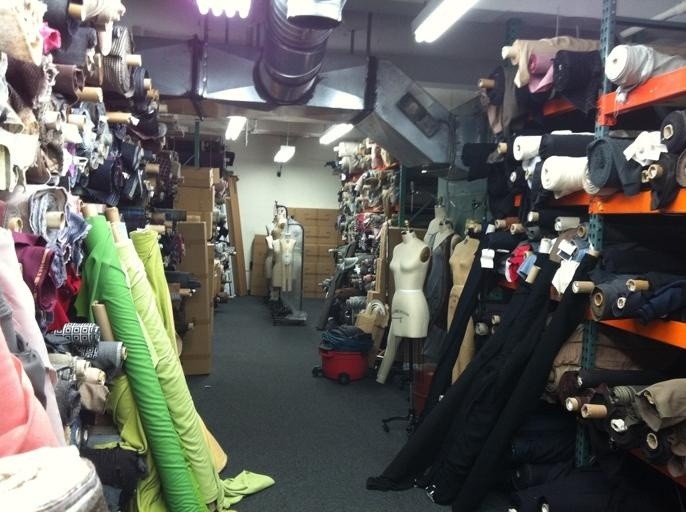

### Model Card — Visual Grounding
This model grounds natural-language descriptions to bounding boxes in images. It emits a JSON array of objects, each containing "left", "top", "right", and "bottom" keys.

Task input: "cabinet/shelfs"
[{"left": 491, "top": 0, "right": 686, "bottom": 512}]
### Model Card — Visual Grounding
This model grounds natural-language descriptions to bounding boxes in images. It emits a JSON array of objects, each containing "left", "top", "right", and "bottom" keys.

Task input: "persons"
[
  {"left": 391, "top": 226, "right": 431, "bottom": 339},
  {"left": 424, "top": 201, "right": 455, "bottom": 243},
  {"left": 420, "top": 217, "right": 460, "bottom": 370},
  {"left": 264, "top": 206, "right": 304, "bottom": 296},
  {"left": 446, "top": 231, "right": 481, "bottom": 387}
]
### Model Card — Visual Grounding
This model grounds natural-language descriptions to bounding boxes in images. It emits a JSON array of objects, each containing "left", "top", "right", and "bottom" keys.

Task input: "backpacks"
[{"left": 318, "top": 324, "right": 375, "bottom": 353}]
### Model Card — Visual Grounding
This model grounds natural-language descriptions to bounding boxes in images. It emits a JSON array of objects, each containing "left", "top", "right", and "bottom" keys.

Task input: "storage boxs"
[
  {"left": 165, "top": 166, "right": 219, "bottom": 374},
  {"left": 366, "top": 291, "right": 394, "bottom": 304},
  {"left": 380, "top": 227, "right": 427, "bottom": 260},
  {"left": 375, "top": 259, "right": 396, "bottom": 292},
  {"left": 249, "top": 208, "right": 345, "bottom": 298}
]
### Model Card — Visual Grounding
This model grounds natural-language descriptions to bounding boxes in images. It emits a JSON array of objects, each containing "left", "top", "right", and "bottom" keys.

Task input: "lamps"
[
  {"left": 221, "top": 103, "right": 247, "bottom": 140},
  {"left": 411, "top": 0, "right": 481, "bottom": 45},
  {"left": 272, "top": 125, "right": 296, "bottom": 163},
  {"left": 319, "top": 122, "right": 354, "bottom": 145}
]
